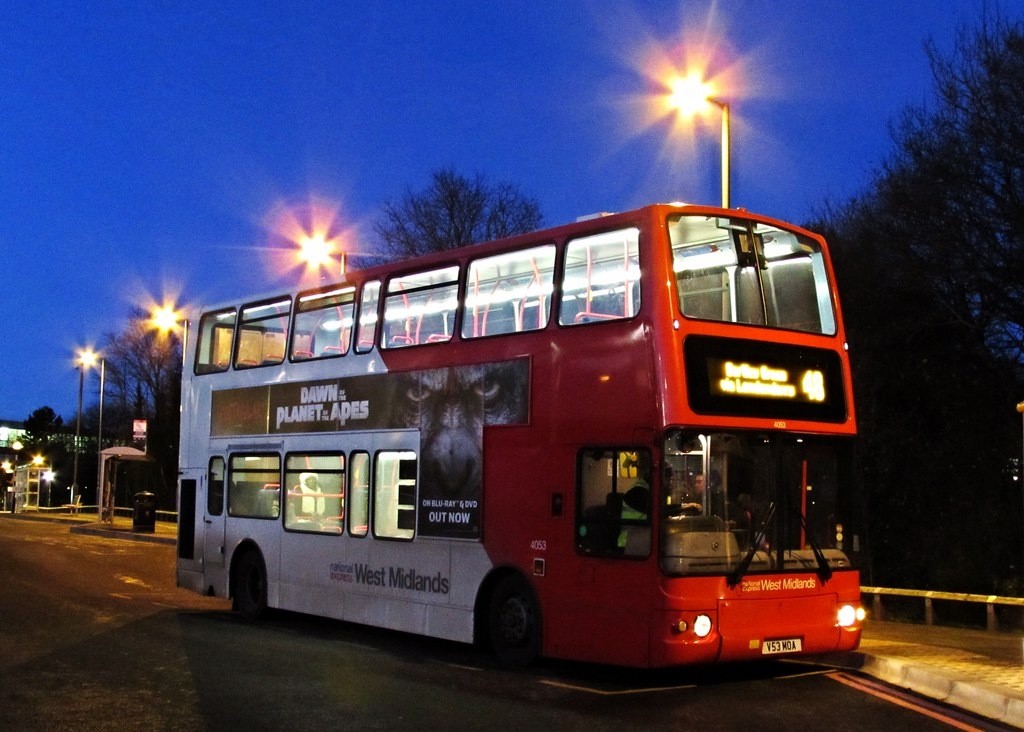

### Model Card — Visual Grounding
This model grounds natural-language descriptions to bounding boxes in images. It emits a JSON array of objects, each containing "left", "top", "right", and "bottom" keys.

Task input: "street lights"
[
  {"left": 80, "top": 350, "right": 105, "bottom": 515},
  {"left": 12, "top": 442, "right": 24, "bottom": 513},
  {"left": 665, "top": 72, "right": 730, "bottom": 570}
]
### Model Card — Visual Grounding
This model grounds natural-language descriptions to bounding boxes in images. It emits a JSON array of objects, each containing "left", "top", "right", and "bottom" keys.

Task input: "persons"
[
  {"left": 680, "top": 469, "right": 749, "bottom": 552},
  {"left": 616, "top": 458, "right": 703, "bottom": 557},
  {"left": 289, "top": 472, "right": 328, "bottom": 524}
]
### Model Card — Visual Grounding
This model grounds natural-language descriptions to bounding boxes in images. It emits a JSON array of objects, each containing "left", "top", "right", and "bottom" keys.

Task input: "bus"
[{"left": 175, "top": 205, "right": 865, "bottom": 673}]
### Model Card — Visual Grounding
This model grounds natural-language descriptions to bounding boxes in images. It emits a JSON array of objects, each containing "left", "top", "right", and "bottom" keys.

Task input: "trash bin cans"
[{"left": 132, "top": 491, "right": 156, "bottom": 533}]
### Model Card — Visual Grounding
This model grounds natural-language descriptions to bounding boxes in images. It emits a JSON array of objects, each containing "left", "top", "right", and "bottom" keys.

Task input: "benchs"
[
  {"left": 574, "top": 312, "right": 623, "bottom": 324},
  {"left": 248, "top": 484, "right": 367, "bottom": 535},
  {"left": 61, "top": 495, "right": 81, "bottom": 514},
  {"left": 240, "top": 335, "right": 452, "bottom": 365}
]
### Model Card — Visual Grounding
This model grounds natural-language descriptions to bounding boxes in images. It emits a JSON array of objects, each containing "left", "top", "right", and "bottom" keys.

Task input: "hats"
[{"left": 663, "top": 460, "right": 672, "bottom": 477}]
